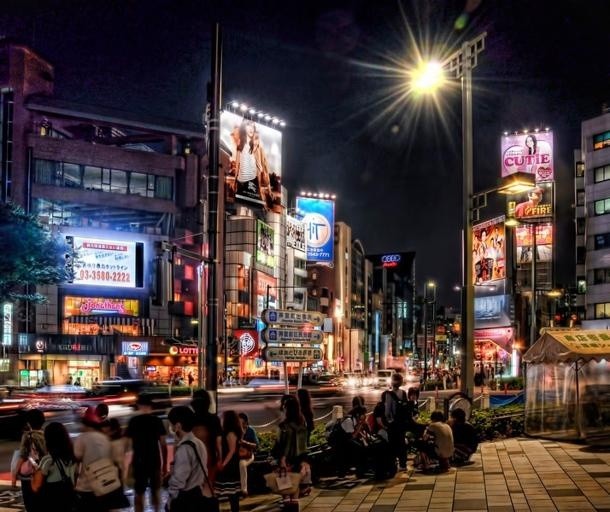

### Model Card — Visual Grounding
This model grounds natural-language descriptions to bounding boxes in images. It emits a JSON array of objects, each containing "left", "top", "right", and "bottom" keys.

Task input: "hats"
[{"left": 137, "top": 394, "right": 154, "bottom": 406}]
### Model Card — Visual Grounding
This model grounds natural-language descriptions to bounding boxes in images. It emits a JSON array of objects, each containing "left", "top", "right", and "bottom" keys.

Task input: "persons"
[
  {"left": 232, "top": 118, "right": 270, "bottom": 208},
  {"left": 272, "top": 388, "right": 316, "bottom": 512},
  {"left": 326, "top": 368, "right": 478, "bottom": 479},
  {"left": 516, "top": 186, "right": 543, "bottom": 218},
  {"left": 10, "top": 390, "right": 261, "bottom": 512},
  {"left": 472, "top": 227, "right": 505, "bottom": 279},
  {"left": 519, "top": 134, "right": 540, "bottom": 174}
]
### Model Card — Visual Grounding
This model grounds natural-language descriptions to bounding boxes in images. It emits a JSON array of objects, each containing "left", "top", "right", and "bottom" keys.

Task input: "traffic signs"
[
  {"left": 262, "top": 347, "right": 324, "bottom": 362},
  {"left": 261, "top": 328, "right": 324, "bottom": 344},
  {"left": 262, "top": 309, "right": 325, "bottom": 326}
]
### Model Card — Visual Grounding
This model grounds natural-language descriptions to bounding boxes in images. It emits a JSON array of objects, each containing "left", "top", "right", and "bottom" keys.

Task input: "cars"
[{"left": 372, "top": 369, "right": 397, "bottom": 389}]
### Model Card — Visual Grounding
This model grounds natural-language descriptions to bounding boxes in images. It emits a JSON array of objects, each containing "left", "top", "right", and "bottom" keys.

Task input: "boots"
[{"left": 282, "top": 497, "right": 299, "bottom": 511}]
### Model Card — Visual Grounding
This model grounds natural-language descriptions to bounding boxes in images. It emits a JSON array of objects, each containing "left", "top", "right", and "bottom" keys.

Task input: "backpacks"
[{"left": 387, "top": 391, "right": 413, "bottom": 428}]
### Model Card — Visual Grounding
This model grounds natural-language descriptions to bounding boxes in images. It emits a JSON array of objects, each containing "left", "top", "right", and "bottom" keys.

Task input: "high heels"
[{"left": 300, "top": 488, "right": 311, "bottom": 497}]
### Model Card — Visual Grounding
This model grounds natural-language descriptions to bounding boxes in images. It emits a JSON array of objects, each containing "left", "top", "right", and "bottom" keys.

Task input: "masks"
[{"left": 168, "top": 426, "right": 177, "bottom": 438}]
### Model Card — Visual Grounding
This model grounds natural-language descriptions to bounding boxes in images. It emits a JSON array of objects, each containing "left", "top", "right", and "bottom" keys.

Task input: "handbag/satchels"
[
  {"left": 85, "top": 459, "right": 122, "bottom": 497},
  {"left": 275, "top": 476, "right": 292, "bottom": 491},
  {"left": 39, "top": 477, "right": 77, "bottom": 510},
  {"left": 325, "top": 416, "right": 356, "bottom": 442},
  {"left": 239, "top": 447, "right": 252, "bottom": 459},
  {"left": 171, "top": 494, "right": 219, "bottom": 511},
  {"left": 11, "top": 450, "right": 41, "bottom": 481},
  {"left": 264, "top": 472, "right": 300, "bottom": 494}
]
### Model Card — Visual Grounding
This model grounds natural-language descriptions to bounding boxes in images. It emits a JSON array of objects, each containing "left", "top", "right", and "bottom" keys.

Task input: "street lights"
[
  {"left": 423, "top": 279, "right": 438, "bottom": 386},
  {"left": 410, "top": 32, "right": 536, "bottom": 400}
]
